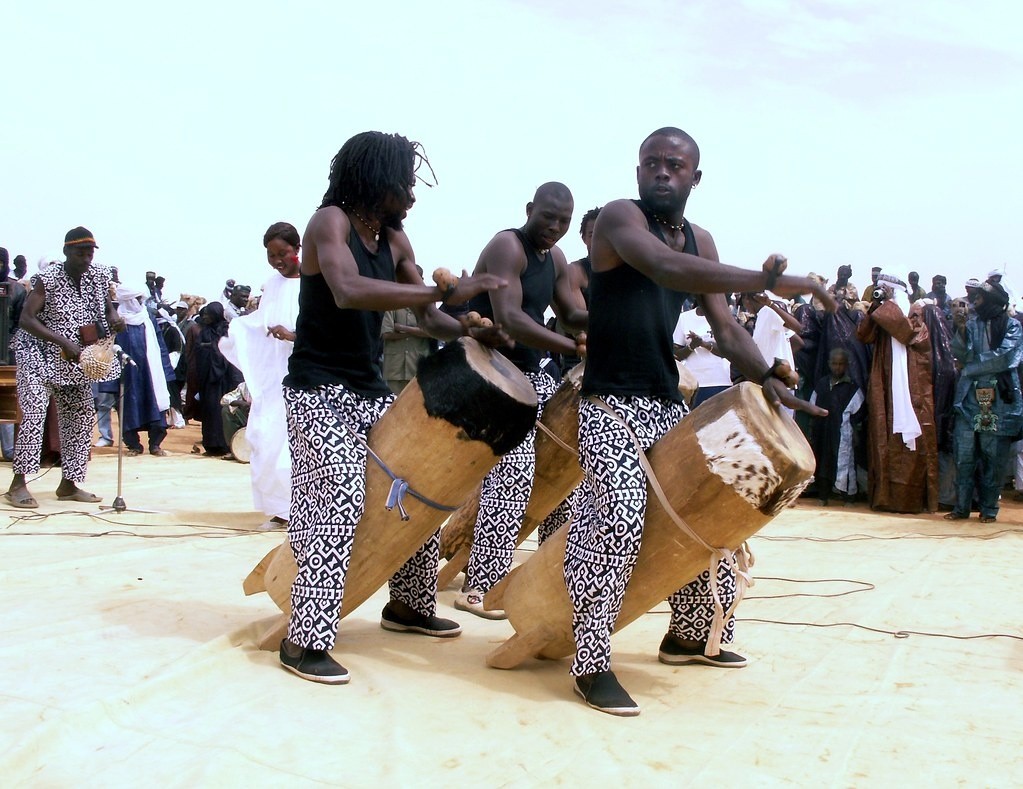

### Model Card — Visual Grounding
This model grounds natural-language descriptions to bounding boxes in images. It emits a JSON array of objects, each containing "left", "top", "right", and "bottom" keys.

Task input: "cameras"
[{"left": 873, "top": 286, "right": 894, "bottom": 299}]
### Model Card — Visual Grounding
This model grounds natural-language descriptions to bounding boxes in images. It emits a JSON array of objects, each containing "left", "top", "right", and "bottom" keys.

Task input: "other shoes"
[
  {"left": 255, "top": 517, "right": 287, "bottom": 531},
  {"left": 978, "top": 514, "right": 997, "bottom": 523},
  {"left": 221, "top": 452, "right": 234, "bottom": 460},
  {"left": 944, "top": 513, "right": 969, "bottom": 520},
  {"left": 820, "top": 499, "right": 856, "bottom": 508},
  {"left": 126, "top": 445, "right": 167, "bottom": 456}
]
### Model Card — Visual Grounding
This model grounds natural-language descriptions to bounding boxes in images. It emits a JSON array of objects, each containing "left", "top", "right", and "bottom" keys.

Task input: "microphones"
[{"left": 112, "top": 344, "right": 136, "bottom": 366}]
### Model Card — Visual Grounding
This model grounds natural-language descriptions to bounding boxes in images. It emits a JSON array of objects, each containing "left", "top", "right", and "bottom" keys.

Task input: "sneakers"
[
  {"left": 454, "top": 587, "right": 507, "bottom": 620},
  {"left": 381, "top": 602, "right": 462, "bottom": 638},
  {"left": 279, "top": 638, "right": 351, "bottom": 684},
  {"left": 93, "top": 438, "right": 114, "bottom": 447},
  {"left": 658, "top": 633, "right": 747, "bottom": 668},
  {"left": 575, "top": 670, "right": 641, "bottom": 717}
]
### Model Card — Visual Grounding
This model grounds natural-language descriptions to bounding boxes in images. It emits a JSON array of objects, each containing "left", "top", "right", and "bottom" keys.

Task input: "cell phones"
[{"left": 958, "top": 301, "right": 965, "bottom": 315}]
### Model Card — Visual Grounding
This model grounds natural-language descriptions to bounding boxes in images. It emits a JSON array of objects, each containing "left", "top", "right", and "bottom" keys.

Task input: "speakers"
[{"left": 0, "top": 282, "right": 10, "bottom": 366}]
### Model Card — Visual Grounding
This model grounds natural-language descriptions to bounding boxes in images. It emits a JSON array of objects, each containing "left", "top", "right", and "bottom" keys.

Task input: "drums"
[{"left": 241, "top": 335, "right": 816, "bottom": 671}]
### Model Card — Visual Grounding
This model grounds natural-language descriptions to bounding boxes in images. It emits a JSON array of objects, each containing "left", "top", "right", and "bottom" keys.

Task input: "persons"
[
  {"left": 29, "top": 258, "right": 271, "bottom": 465},
  {"left": 672, "top": 292, "right": 738, "bottom": 411},
  {"left": 7, "top": 255, "right": 35, "bottom": 291},
  {"left": 553, "top": 208, "right": 603, "bottom": 372},
  {"left": 563, "top": 127, "right": 839, "bottom": 716},
  {"left": 4, "top": 227, "right": 126, "bottom": 508},
  {"left": 379, "top": 265, "right": 440, "bottom": 397},
  {"left": 278, "top": 131, "right": 516, "bottom": 684},
  {"left": 0, "top": 247, "right": 26, "bottom": 463},
  {"left": 453, "top": 183, "right": 582, "bottom": 620},
  {"left": 729, "top": 265, "right": 1023, "bottom": 523},
  {"left": 219, "top": 222, "right": 304, "bottom": 524}
]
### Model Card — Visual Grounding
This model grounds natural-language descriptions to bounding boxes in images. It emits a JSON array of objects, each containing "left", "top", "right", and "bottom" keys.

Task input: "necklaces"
[
  {"left": 346, "top": 203, "right": 382, "bottom": 241},
  {"left": 651, "top": 212, "right": 685, "bottom": 230},
  {"left": 524, "top": 225, "right": 550, "bottom": 255}
]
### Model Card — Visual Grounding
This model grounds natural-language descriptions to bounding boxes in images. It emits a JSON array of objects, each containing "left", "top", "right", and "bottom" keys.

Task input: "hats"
[
  {"left": 978, "top": 275, "right": 1009, "bottom": 305},
  {"left": 65, "top": 226, "right": 99, "bottom": 248}
]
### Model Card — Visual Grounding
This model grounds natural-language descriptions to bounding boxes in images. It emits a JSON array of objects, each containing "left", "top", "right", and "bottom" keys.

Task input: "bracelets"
[
  {"left": 709, "top": 341, "right": 714, "bottom": 352},
  {"left": 576, "top": 332, "right": 588, "bottom": 358},
  {"left": 760, "top": 356, "right": 800, "bottom": 385},
  {"left": 762, "top": 253, "right": 789, "bottom": 290},
  {"left": 688, "top": 344, "right": 694, "bottom": 352},
  {"left": 432, "top": 269, "right": 458, "bottom": 303},
  {"left": 461, "top": 311, "right": 493, "bottom": 338}
]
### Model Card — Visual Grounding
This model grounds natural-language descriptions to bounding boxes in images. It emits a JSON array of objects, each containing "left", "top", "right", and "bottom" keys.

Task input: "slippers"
[
  {"left": 56, "top": 490, "right": 104, "bottom": 502},
  {"left": 4, "top": 487, "right": 38, "bottom": 508}
]
